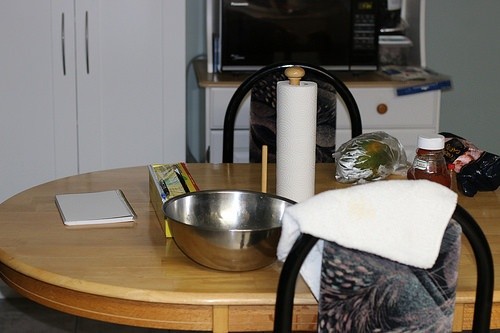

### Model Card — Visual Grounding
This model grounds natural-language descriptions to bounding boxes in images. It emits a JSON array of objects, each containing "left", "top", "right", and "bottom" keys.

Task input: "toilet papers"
[{"left": 274, "top": 80, "right": 318, "bottom": 203}]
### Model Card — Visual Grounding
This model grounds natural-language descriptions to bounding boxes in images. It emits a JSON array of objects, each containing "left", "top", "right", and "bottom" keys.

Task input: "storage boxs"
[{"left": 149, "top": 162, "right": 201, "bottom": 238}]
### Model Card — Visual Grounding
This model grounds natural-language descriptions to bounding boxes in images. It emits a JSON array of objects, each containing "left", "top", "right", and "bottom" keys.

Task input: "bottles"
[
  {"left": 405, "top": 133, "right": 451, "bottom": 193},
  {"left": 445, "top": 164, "right": 458, "bottom": 198}
]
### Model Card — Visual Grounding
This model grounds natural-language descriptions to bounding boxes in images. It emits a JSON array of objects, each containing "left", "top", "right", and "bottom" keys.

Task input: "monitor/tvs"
[{"left": 217, "top": 0, "right": 382, "bottom": 77}]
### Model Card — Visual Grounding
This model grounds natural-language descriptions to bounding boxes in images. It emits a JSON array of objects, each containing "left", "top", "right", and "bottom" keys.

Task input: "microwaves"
[{"left": 215, "top": 0, "right": 384, "bottom": 74}]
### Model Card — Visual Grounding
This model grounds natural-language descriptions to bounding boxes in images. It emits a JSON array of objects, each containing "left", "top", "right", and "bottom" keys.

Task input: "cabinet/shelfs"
[
  {"left": 200, "top": 66, "right": 453, "bottom": 162},
  {"left": 0, "top": 0, "right": 206, "bottom": 205}
]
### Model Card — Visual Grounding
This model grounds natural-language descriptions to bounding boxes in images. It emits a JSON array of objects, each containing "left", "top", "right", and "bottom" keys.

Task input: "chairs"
[
  {"left": 221, "top": 59, "right": 362, "bottom": 163},
  {"left": 271, "top": 179, "right": 496, "bottom": 333}
]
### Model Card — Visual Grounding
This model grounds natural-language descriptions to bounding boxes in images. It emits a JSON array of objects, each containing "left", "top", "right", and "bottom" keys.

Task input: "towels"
[{"left": 275, "top": 179, "right": 459, "bottom": 301}]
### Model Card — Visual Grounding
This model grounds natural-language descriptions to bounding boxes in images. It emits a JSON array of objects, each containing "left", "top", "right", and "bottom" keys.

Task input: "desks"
[{"left": 0, "top": 162, "right": 500, "bottom": 333}]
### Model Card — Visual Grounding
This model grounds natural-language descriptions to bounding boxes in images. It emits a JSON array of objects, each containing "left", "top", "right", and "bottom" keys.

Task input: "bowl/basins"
[{"left": 162, "top": 189, "right": 300, "bottom": 271}]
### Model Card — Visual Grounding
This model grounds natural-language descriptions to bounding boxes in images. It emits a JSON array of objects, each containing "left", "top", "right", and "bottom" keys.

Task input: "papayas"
[{"left": 337, "top": 131, "right": 403, "bottom": 183}]
